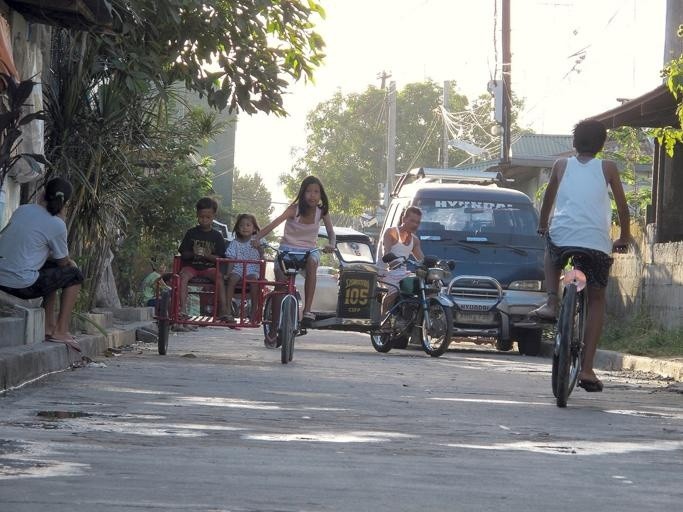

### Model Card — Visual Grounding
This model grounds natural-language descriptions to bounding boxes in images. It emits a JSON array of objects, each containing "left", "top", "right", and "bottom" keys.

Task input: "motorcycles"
[{"left": 369, "top": 254, "right": 460, "bottom": 355}]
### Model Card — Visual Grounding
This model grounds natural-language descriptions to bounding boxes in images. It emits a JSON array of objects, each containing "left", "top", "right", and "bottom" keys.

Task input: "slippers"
[
  {"left": 303, "top": 312, "right": 316, "bottom": 320},
  {"left": 264, "top": 335, "right": 278, "bottom": 349},
  {"left": 528, "top": 311, "right": 557, "bottom": 324},
  {"left": 576, "top": 380, "right": 603, "bottom": 392},
  {"left": 48, "top": 339, "right": 83, "bottom": 355}
]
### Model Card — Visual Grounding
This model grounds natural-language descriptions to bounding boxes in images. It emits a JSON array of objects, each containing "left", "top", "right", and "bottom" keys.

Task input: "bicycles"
[
  {"left": 538, "top": 228, "right": 589, "bottom": 408},
  {"left": 257, "top": 244, "right": 338, "bottom": 367}
]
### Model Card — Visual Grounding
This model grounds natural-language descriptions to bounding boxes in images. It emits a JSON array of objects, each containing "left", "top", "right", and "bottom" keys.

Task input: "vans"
[{"left": 372, "top": 168, "right": 553, "bottom": 358}]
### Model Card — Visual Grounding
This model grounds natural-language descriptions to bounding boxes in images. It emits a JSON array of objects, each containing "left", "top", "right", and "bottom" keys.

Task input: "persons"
[
  {"left": 250, "top": 175, "right": 336, "bottom": 350},
  {"left": 223, "top": 212, "right": 269, "bottom": 324},
  {"left": 177, "top": 197, "right": 232, "bottom": 321},
  {"left": 375, "top": 205, "right": 425, "bottom": 321},
  {"left": 140, "top": 263, "right": 171, "bottom": 306},
  {"left": 164, "top": 274, "right": 173, "bottom": 285},
  {"left": 0, "top": 175, "right": 83, "bottom": 356},
  {"left": 527, "top": 117, "right": 631, "bottom": 391}
]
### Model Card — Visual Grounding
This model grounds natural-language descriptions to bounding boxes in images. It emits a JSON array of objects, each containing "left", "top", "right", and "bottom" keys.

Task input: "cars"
[{"left": 312, "top": 226, "right": 379, "bottom": 332}]
